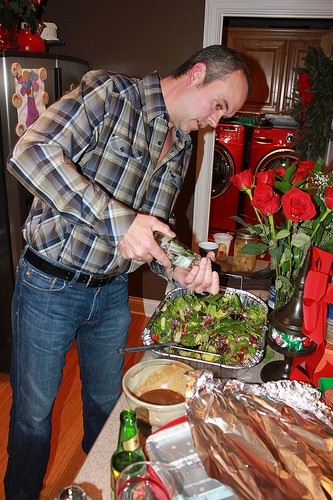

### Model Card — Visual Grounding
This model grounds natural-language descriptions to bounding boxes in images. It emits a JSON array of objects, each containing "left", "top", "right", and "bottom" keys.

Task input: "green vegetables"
[{"left": 153, "top": 291, "right": 267, "bottom": 364}]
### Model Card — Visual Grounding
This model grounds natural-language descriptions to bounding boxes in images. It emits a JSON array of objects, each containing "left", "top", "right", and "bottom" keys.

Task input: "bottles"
[
  {"left": 110, "top": 409, "right": 146, "bottom": 500},
  {"left": 152, "top": 229, "right": 228, "bottom": 287},
  {"left": 54, "top": 485, "right": 92, "bottom": 500},
  {"left": 267, "top": 270, "right": 277, "bottom": 312},
  {"left": 233, "top": 227, "right": 259, "bottom": 272}
]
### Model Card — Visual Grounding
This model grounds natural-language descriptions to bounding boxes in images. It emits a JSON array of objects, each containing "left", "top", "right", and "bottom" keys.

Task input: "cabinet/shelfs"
[{"left": 222, "top": 27, "right": 333, "bottom": 116}]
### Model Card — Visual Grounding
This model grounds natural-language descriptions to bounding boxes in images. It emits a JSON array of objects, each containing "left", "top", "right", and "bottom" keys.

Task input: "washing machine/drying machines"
[
  {"left": 241, "top": 115, "right": 304, "bottom": 234},
  {"left": 208, "top": 115, "right": 246, "bottom": 233}
]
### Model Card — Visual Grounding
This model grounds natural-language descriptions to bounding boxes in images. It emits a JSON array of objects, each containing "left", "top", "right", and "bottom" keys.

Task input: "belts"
[{"left": 24, "top": 249, "right": 118, "bottom": 288}]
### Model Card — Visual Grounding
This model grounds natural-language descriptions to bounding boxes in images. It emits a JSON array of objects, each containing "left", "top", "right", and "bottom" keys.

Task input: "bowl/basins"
[{"left": 122, "top": 358, "right": 194, "bottom": 427}]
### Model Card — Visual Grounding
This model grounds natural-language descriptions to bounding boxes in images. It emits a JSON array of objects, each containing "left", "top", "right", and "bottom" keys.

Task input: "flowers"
[{"left": 230, "top": 156, "right": 333, "bottom": 308}]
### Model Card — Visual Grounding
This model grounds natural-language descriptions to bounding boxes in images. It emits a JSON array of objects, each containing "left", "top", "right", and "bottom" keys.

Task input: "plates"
[{"left": 144, "top": 421, "right": 239, "bottom": 500}]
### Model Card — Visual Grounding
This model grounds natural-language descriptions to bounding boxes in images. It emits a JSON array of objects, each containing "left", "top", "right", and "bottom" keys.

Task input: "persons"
[{"left": 4, "top": 44, "right": 252, "bottom": 500}]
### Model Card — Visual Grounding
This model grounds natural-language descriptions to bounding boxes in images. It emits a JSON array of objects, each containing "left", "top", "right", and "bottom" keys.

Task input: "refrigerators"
[{"left": 0, "top": 51, "right": 88, "bottom": 374}]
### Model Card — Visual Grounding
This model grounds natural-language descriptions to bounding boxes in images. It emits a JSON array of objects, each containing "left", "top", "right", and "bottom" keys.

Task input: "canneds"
[{"left": 233, "top": 229, "right": 257, "bottom": 272}]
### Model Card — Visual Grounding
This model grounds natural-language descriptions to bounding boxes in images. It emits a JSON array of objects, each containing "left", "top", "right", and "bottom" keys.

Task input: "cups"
[
  {"left": 197, "top": 241, "right": 219, "bottom": 259},
  {"left": 213, "top": 233, "right": 234, "bottom": 262},
  {"left": 115, "top": 461, "right": 176, "bottom": 500}
]
